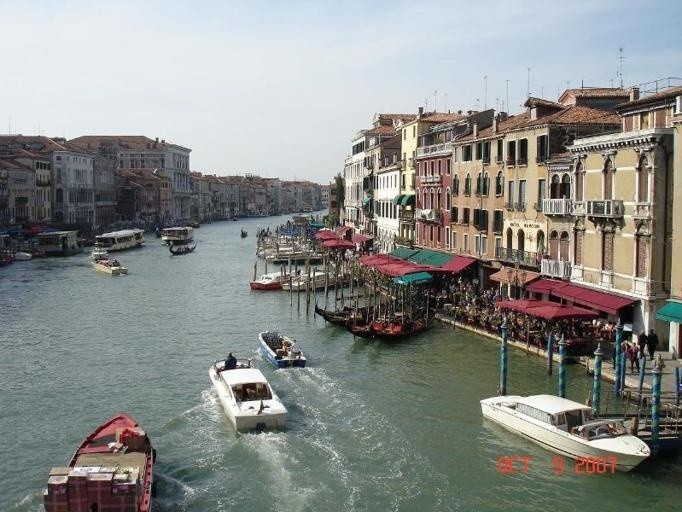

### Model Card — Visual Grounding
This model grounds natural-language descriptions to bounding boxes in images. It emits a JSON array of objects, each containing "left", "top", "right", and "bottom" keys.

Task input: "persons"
[
  {"left": 290, "top": 339, "right": 300, "bottom": 354},
  {"left": 615, "top": 329, "right": 665, "bottom": 374},
  {"left": 223, "top": 351, "right": 237, "bottom": 371},
  {"left": 422, "top": 273, "right": 617, "bottom": 339}
]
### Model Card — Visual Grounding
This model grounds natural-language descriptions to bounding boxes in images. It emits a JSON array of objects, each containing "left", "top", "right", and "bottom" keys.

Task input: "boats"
[
  {"left": 478, "top": 393, "right": 652, "bottom": 474},
  {"left": 208, "top": 357, "right": 289, "bottom": 438},
  {"left": 42, "top": 413, "right": 157, "bottom": 512},
  {"left": 29, "top": 224, "right": 198, "bottom": 277},
  {"left": 251, "top": 224, "right": 437, "bottom": 342},
  {"left": 258, "top": 329, "right": 307, "bottom": 369}
]
